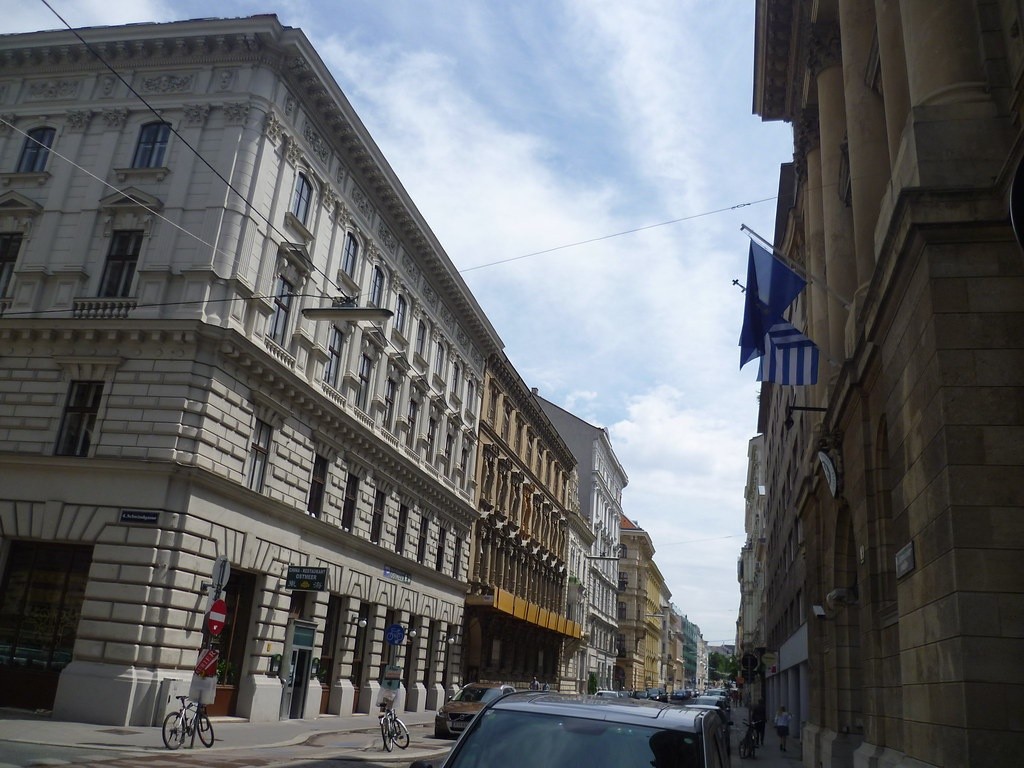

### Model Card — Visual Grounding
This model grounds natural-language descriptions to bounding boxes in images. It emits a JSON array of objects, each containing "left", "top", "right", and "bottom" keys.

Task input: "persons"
[
  {"left": 543, "top": 680, "right": 550, "bottom": 690},
  {"left": 732, "top": 686, "right": 743, "bottom": 707},
  {"left": 774, "top": 705, "right": 792, "bottom": 752},
  {"left": 530, "top": 676, "right": 541, "bottom": 690},
  {"left": 752, "top": 698, "right": 767, "bottom": 747}
]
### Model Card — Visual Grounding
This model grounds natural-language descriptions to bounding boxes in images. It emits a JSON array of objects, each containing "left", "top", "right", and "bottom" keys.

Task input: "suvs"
[{"left": 435, "top": 682, "right": 516, "bottom": 739}]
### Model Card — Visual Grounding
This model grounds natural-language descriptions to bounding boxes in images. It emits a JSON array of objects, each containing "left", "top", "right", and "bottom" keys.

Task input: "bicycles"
[
  {"left": 162, "top": 696, "right": 214, "bottom": 750},
  {"left": 739, "top": 718, "right": 762, "bottom": 758},
  {"left": 377, "top": 701, "right": 410, "bottom": 752}
]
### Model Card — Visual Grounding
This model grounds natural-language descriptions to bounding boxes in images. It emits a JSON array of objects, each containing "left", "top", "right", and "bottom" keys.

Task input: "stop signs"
[{"left": 208, "top": 599, "right": 227, "bottom": 635}]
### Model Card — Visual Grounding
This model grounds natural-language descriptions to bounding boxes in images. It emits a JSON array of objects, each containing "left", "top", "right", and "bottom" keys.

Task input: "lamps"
[
  {"left": 300, "top": 298, "right": 396, "bottom": 320},
  {"left": 443, "top": 633, "right": 455, "bottom": 644},
  {"left": 352, "top": 615, "right": 368, "bottom": 627},
  {"left": 403, "top": 627, "right": 418, "bottom": 638}
]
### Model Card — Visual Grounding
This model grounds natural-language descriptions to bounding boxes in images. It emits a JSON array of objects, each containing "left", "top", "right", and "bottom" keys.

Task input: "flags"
[
  {"left": 739, "top": 240, "right": 807, "bottom": 370},
  {"left": 744, "top": 292, "right": 818, "bottom": 386}
]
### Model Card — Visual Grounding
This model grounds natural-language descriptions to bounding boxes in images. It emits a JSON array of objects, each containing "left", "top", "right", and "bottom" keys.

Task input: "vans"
[{"left": 440, "top": 689, "right": 731, "bottom": 768}]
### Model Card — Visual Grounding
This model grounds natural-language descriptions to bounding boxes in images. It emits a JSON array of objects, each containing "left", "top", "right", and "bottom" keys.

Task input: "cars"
[
  {"left": 686, "top": 691, "right": 734, "bottom": 737},
  {"left": 595, "top": 688, "right": 670, "bottom": 702},
  {"left": 671, "top": 687, "right": 701, "bottom": 700}
]
